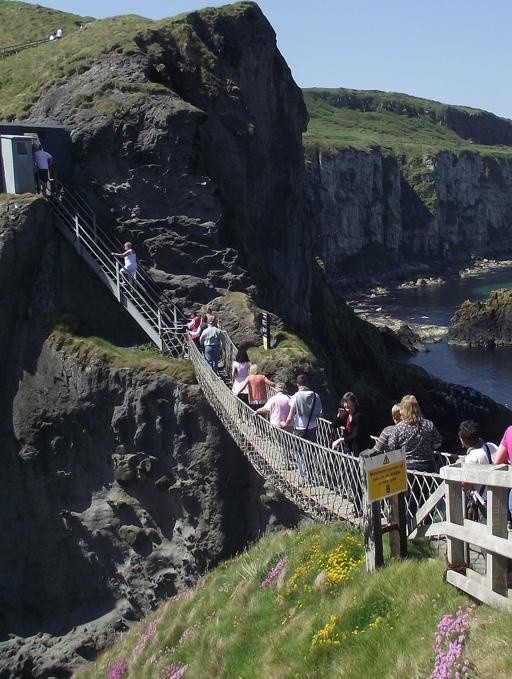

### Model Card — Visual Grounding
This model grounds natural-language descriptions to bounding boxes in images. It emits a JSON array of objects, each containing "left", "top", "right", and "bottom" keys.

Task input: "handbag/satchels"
[
  {"left": 292, "top": 429, "right": 307, "bottom": 439},
  {"left": 466, "top": 499, "right": 479, "bottom": 522}
]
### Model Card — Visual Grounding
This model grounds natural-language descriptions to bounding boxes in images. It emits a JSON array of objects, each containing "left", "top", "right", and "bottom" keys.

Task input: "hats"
[
  {"left": 277, "top": 383, "right": 289, "bottom": 394},
  {"left": 248, "top": 365, "right": 261, "bottom": 376}
]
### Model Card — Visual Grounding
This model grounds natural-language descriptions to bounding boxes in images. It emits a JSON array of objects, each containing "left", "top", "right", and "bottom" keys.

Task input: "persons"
[
  {"left": 231, "top": 344, "right": 251, "bottom": 405},
  {"left": 331, "top": 391, "right": 369, "bottom": 500},
  {"left": 55, "top": 28, "right": 63, "bottom": 39},
  {"left": 360, "top": 394, "right": 512, "bottom": 533},
  {"left": 186, "top": 306, "right": 226, "bottom": 377},
  {"left": 253, "top": 382, "right": 295, "bottom": 468},
  {"left": 111, "top": 242, "right": 137, "bottom": 292},
  {"left": 234, "top": 364, "right": 276, "bottom": 428},
  {"left": 33, "top": 144, "right": 53, "bottom": 192},
  {"left": 280, "top": 373, "right": 323, "bottom": 482}
]
